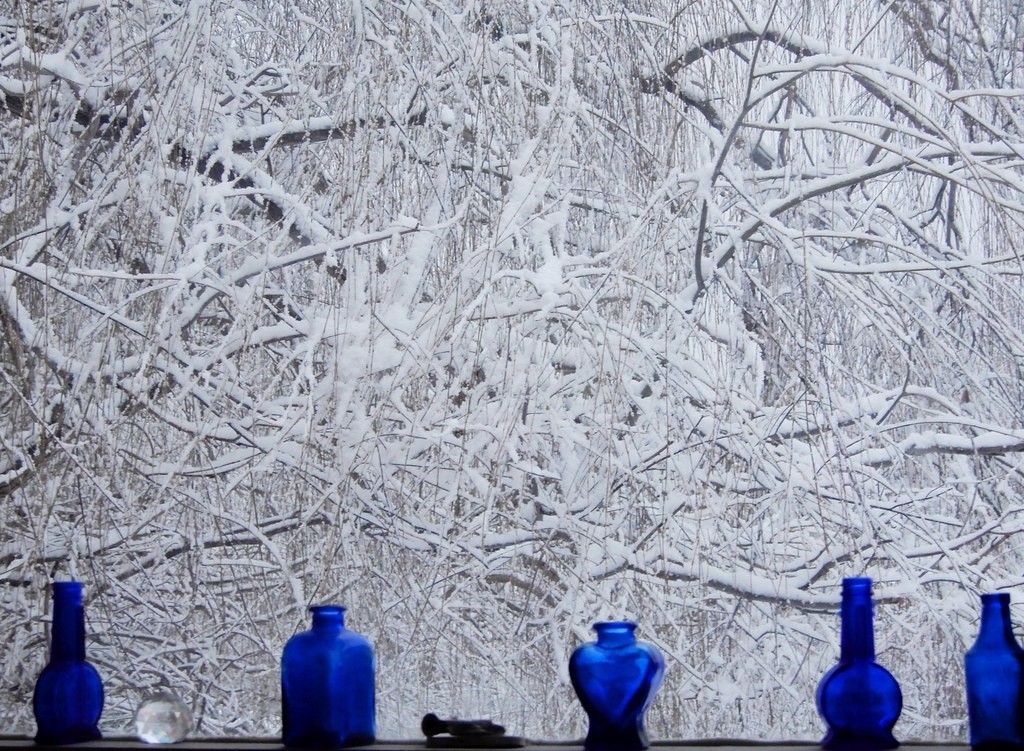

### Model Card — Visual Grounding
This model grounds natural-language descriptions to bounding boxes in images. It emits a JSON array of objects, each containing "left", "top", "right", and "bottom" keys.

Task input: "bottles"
[
  {"left": 281, "top": 606, "right": 377, "bottom": 750},
  {"left": 963, "top": 593, "right": 1024, "bottom": 751},
  {"left": 815, "top": 577, "right": 903, "bottom": 751},
  {"left": 32, "top": 581, "right": 105, "bottom": 744}
]
